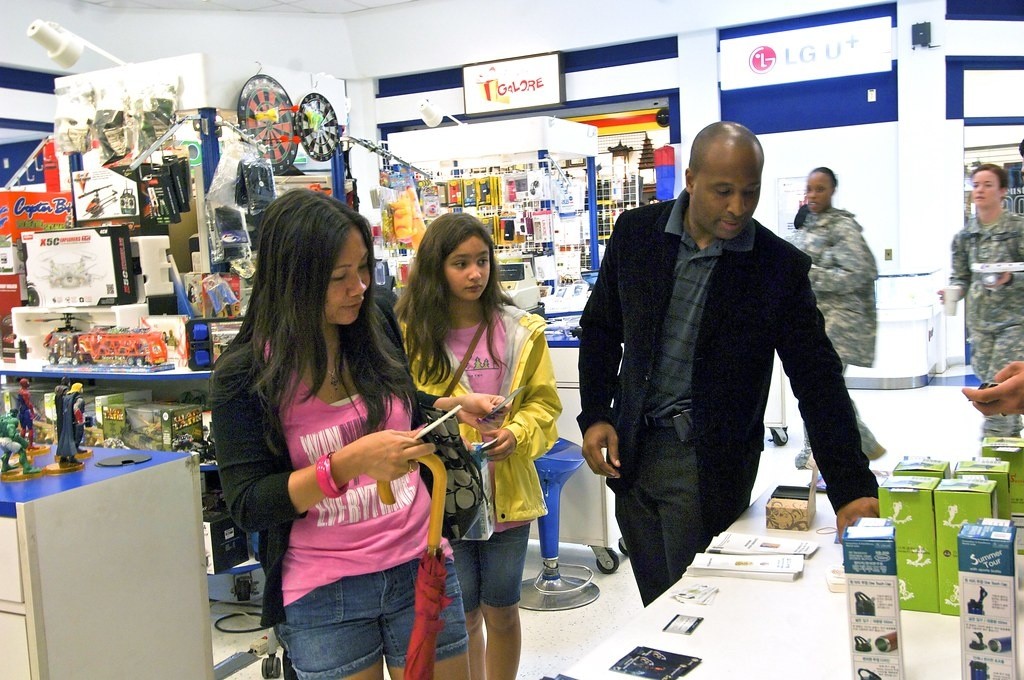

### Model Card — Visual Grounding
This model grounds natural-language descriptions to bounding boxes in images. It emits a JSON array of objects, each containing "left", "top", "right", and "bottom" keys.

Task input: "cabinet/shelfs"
[
  {"left": 528, "top": 333, "right": 628, "bottom": 573},
  {"left": 0, "top": 362, "right": 266, "bottom": 605},
  {"left": 0, "top": 444, "right": 215, "bottom": 680}
]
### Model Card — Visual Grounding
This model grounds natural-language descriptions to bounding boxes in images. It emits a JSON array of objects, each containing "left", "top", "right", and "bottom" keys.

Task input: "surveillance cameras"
[{"left": 656, "top": 108, "right": 670, "bottom": 127}]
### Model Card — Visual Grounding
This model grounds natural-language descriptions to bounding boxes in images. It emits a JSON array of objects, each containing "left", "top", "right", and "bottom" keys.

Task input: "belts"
[{"left": 641, "top": 414, "right": 672, "bottom": 428}]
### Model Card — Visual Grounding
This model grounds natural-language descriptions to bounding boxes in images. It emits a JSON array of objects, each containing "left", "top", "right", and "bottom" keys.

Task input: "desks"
[{"left": 565, "top": 463, "right": 960, "bottom": 680}]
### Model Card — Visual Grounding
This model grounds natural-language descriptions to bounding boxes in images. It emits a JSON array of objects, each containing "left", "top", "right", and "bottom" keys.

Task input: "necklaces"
[{"left": 326, "top": 367, "right": 340, "bottom": 392}]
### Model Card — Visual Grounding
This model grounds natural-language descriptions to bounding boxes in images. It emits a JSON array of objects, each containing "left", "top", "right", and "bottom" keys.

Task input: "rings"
[
  {"left": 999, "top": 412, "right": 1008, "bottom": 417},
  {"left": 408, "top": 464, "right": 414, "bottom": 473}
]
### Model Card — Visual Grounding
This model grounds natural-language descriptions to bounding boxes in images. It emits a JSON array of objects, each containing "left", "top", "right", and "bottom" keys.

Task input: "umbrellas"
[{"left": 376, "top": 453, "right": 454, "bottom": 680}]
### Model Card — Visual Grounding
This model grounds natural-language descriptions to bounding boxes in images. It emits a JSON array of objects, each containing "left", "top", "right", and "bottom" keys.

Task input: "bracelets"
[{"left": 314, "top": 451, "right": 350, "bottom": 499}]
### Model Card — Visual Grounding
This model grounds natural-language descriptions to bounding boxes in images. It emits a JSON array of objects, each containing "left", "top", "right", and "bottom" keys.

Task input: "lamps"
[
  {"left": 608, "top": 140, "right": 633, "bottom": 181},
  {"left": 638, "top": 131, "right": 657, "bottom": 187},
  {"left": 27, "top": 18, "right": 131, "bottom": 70},
  {"left": 419, "top": 99, "right": 468, "bottom": 127}
]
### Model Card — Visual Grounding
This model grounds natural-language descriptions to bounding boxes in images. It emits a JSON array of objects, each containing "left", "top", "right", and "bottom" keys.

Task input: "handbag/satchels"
[{"left": 373, "top": 297, "right": 484, "bottom": 542}]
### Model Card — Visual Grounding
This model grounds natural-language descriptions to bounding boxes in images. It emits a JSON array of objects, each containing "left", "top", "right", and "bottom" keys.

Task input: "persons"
[
  {"left": 0, "top": 409, "right": 42, "bottom": 474},
  {"left": 936, "top": 163, "right": 1024, "bottom": 440},
  {"left": 202, "top": 187, "right": 473, "bottom": 680},
  {"left": 54, "top": 377, "right": 88, "bottom": 453},
  {"left": 1018, "top": 139, "right": 1024, "bottom": 173},
  {"left": 794, "top": 166, "right": 886, "bottom": 468},
  {"left": 393, "top": 215, "right": 566, "bottom": 680},
  {"left": 54, "top": 382, "right": 85, "bottom": 467},
  {"left": 575, "top": 119, "right": 881, "bottom": 605},
  {"left": 961, "top": 359, "right": 1024, "bottom": 416},
  {"left": 14, "top": 378, "right": 40, "bottom": 451}
]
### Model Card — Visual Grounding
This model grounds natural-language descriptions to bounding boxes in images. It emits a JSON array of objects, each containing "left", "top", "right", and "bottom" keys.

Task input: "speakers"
[{"left": 912, "top": 21, "right": 931, "bottom": 45}]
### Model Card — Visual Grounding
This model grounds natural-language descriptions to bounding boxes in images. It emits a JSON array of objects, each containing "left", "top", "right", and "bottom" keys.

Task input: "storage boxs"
[
  {"left": 179, "top": 272, "right": 247, "bottom": 318},
  {"left": 435, "top": 176, "right": 525, "bottom": 245},
  {"left": 201, "top": 473, "right": 249, "bottom": 575},
  {"left": 0, "top": 140, "right": 193, "bottom": 368},
  {"left": 4, "top": 386, "right": 212, "bottom": 452},
  {"left": 764, "top": 437, "right": 1024, "bottom": 680}
]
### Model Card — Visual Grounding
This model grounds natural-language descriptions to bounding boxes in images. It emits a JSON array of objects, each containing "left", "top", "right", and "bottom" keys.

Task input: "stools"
[{"left": 518, "top": 438, "right": 600, "bottom": 612}]
